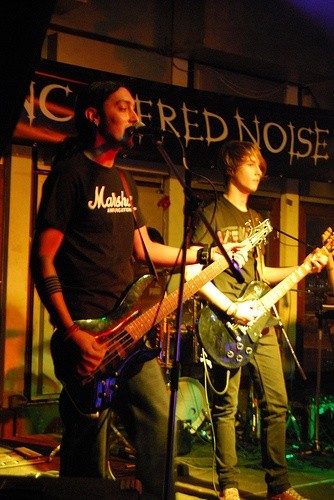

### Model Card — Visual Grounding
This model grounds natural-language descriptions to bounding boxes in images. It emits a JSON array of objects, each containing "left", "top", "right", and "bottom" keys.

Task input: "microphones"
[{"left": 134, "top": 121, "right": 162, "bottom": 145}]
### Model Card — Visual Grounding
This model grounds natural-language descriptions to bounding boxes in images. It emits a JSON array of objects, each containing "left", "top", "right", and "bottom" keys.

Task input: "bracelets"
[
  {"left": 224, "top": 302, "right": 238, "bottom": 318},
  {"left": 62, "top": 323, "right": 80, "bottom": 341},
  {"left": 198, "top": 247, "right": 210, "bottom": 267}
]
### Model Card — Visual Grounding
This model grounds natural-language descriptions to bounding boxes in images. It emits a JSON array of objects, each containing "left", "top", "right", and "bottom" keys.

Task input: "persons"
[
  {"left": 30, "top": 82, "right": 249, "bottom": 500},
  {"left": 184, "top": 141, "right": 328, "bottom": 500}
]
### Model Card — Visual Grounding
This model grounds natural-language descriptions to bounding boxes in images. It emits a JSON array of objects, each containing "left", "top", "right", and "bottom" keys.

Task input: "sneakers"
[{"left": 219, "top": 488, "right": 310, "bottom": 500}]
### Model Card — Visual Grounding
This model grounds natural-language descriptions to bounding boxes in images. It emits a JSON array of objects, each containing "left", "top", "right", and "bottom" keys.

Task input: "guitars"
[
  {"left": 197, "top": 227, "right": 334, "bottom": 369},
  {"left": 49, "top": 218, "right": 273, "bottom": 413}
]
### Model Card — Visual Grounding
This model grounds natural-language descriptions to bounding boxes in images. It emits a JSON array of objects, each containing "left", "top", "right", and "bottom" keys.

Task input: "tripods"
[{"left": 285, "top": 318, "right": 334, "bottom": 459}]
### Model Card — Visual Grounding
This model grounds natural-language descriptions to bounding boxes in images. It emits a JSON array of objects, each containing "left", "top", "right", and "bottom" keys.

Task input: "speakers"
[{"left": 0, "top": 475, "right": 143, "bottom": 500}]
